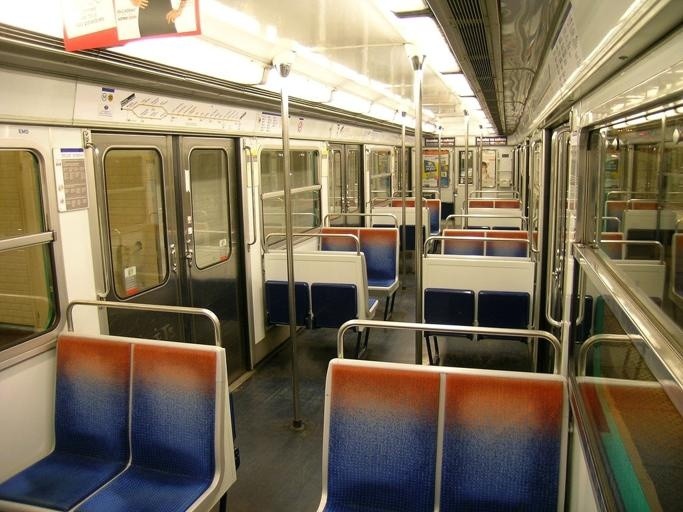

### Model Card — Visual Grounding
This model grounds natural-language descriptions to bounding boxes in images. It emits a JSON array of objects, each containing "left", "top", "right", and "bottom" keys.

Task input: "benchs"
[
  {"left": 316, "top": 319, "right": 570, "bottom": 512},
  {"left": 0, "top": 300, "right": 238, "bottom": 512},
  {"left": 594, "top": 190, "right": 683, "bottom": 330}
]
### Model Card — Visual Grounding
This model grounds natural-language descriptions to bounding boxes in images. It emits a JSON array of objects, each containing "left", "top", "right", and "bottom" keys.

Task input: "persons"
[{"left": 128, "top": 0, "right": 190, "bottom": 38}]
